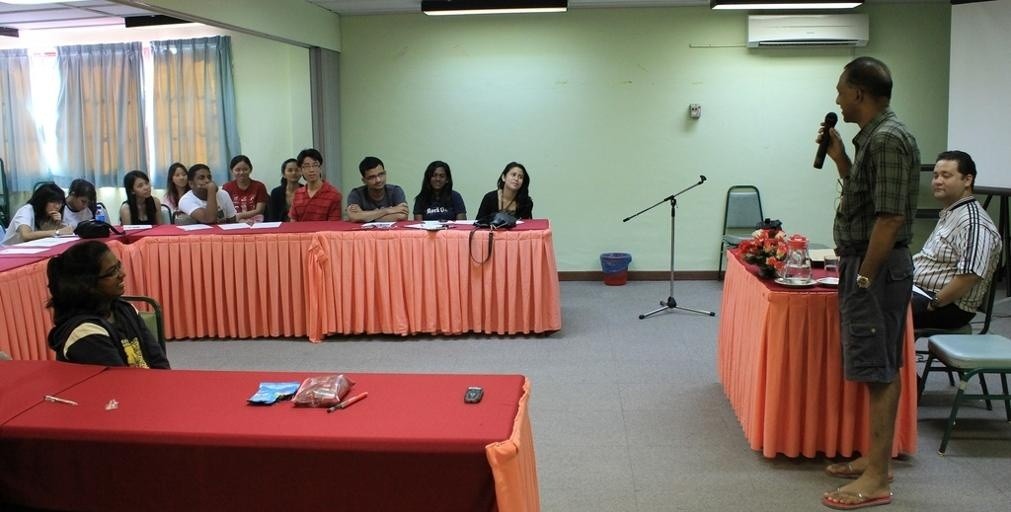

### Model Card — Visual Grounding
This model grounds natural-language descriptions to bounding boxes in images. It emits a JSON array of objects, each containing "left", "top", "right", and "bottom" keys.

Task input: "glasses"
[
  {"left": 364, "top": 171, "right": 385, "bottom": 179},
  {"left": 94, "top": 259, "right": 121, "bottom": 283},
  {"left": 300, "top": 164, "right": 321, "bottom": 170}
]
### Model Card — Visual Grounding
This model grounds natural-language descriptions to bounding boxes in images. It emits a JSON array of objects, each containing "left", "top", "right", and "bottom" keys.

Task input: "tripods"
[{"left": 622, "top": 175, "right": 716, "bottom": 320}]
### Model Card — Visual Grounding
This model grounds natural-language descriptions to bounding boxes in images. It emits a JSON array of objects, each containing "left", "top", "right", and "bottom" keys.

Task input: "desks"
[
  {"left": 1, "top": 225, "right": 155, "bottom": 361},
  {"left": 128, "top": 217, "right": 564, "bottom": 342},
  {"left": 1, "top": 360, "right": 108, "bottom": 420},
  {"left": 719, "top": 247, "right": 918, "bottom": 458},
  {"left": 1, "top": 368, "right": 528, "bottom": 512}
]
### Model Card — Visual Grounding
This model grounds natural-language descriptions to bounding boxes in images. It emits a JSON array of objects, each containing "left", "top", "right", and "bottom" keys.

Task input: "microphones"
[{"left": 813, "top": 112, "right": 838, "bottom": 170}]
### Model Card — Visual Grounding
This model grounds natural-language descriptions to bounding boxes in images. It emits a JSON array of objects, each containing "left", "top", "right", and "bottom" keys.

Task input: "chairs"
[
  {"left": 96, "top": 201, "right": 111, "bottom": 225},
  {"left": 122, "top": 294, "right": 168, "bottom": 357},
  {"left": 720, "top": 185, "right": 764, "bottom": 281},
  {"left": 918, "top": 333, "right": 1011, "bottom": 456},
  {"left": 160, "top": 205, "right": 172, "bottom": 225},
  {"left": 915, "top": 247, "right": 1004, "bottom": 390}
]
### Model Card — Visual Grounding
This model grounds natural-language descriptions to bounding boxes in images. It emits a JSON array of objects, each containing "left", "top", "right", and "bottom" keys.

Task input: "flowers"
[{"left": 737, "top": 218, "right": 789, "bottom": 271}]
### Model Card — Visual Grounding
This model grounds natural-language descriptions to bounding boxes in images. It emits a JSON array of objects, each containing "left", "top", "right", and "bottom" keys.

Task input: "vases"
[{"left": 759, "top": 263, "right": 778, "bottom": 281}]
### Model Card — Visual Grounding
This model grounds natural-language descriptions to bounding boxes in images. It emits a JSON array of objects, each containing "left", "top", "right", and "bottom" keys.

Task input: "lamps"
[
  {"left": 710, "top": 2, "right": 866, "bottom": 13},
  {"left": 418, "top": 0, "right": 568, "bottom": 16}
]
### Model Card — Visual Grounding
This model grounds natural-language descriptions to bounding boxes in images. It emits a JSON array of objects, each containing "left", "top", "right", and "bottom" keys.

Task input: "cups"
[
  {"left": 783, "top": 248, "right": 812, "bottom": 284},
  {"left": 824, "top": 256, "right": 840, "bottom": 280}
]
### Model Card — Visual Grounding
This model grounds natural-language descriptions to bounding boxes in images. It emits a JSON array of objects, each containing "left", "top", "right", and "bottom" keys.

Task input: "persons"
[
  {"left": 162, "top": 163, "right": 191, "bottom": 224},
  {"left": 178, "top": 166, "right": 236, "bottom": 224},
  {"left": 119, "top": 171, "right": 160, "bottom": 225},
  {"left": 914, "top": 151, "right": 1000, "bottom": 332},
  {"left": 1, "top": 183, "right": 65, "bottom": 245},
  {"left": 348, "top": 157, "right": 410, "bottom": 222},
  {"left": 268, "top": 158, "right": 302, "bottom": 222},
  {"left": 820, "top": 57, "right": 920, "bottom": 507},
  {"left": 47, "top": 241, "right": 173, "bottom": 369},
  {"left": 290, "top": 149, "right": 343, "bottom": 221},
  {"left": 414, "top": 160, "right": 466, "bottom": 224},
  {"left": 63, "top": 178, "right": 97, "bottom": 230},
  {"left": 223, "top": 156, "right": 267, "bottom": 221},
  {"left": 475, "top": 163, "right": 533, "bottom": 226}
]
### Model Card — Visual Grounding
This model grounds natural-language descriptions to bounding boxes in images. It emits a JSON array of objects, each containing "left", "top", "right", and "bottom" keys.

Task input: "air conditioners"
[{"left": 746, "top": 13, "right": 869, "bottom": 48}]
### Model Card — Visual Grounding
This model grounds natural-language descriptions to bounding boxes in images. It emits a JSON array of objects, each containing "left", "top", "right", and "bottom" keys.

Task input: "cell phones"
[{"left": 463, "top": 385, "right": 485, "bottom": 405}]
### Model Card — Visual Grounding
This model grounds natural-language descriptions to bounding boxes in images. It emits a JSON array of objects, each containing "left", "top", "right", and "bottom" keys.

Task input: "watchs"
[
  {"left": 929, "top": 294, "right": 944, "bottom": 310},
  {"left": 854, "top": 272, "right": 870, "bottom": 290}
]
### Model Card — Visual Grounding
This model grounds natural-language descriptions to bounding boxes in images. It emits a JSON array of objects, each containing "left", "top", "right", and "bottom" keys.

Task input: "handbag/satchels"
[{"left": 72, "top": 218, "right": 122, "bottom": 240}]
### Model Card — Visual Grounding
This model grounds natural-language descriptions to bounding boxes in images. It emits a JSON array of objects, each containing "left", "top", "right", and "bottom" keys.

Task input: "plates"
[
  {"left": 774, "top": 277, "right": 817, "bottom": 286},
  {"left": 817, "top": 277, "right": 840, "bottom": 285}
]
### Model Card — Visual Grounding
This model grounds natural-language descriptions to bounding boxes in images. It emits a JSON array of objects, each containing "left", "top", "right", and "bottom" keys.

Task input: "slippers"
[
  {"left": 823, "top": 461, "right": 894, "bottom": 483},
  {"left": 819, "top": 487, "right": 895, "bottom": 511}
]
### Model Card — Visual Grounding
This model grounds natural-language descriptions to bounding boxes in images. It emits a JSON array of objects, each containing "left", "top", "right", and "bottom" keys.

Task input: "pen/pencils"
[
  {"left": 68, "top": 223, "right": 69, "bottom": 226},
  {"left": 44, "top": 395, "right": 78, "bottom": 405},
  {"left": 327, "top": 392, "right": 368, "bottom": 413}
]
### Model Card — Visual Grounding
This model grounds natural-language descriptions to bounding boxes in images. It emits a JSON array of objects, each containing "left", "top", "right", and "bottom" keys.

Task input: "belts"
[{"left": 832, "top": 238, "right": 909, "bottom": 258}]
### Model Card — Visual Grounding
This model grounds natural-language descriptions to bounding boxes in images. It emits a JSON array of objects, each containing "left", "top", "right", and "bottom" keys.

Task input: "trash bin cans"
[{"left": 600, "top": 253, "right": 632, "bottom": 286}]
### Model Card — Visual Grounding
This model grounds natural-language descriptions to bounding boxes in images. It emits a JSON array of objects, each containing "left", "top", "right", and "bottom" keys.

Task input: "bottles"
[{"left": 95, "top": 205, "right": 105, "bottom": 222}]
